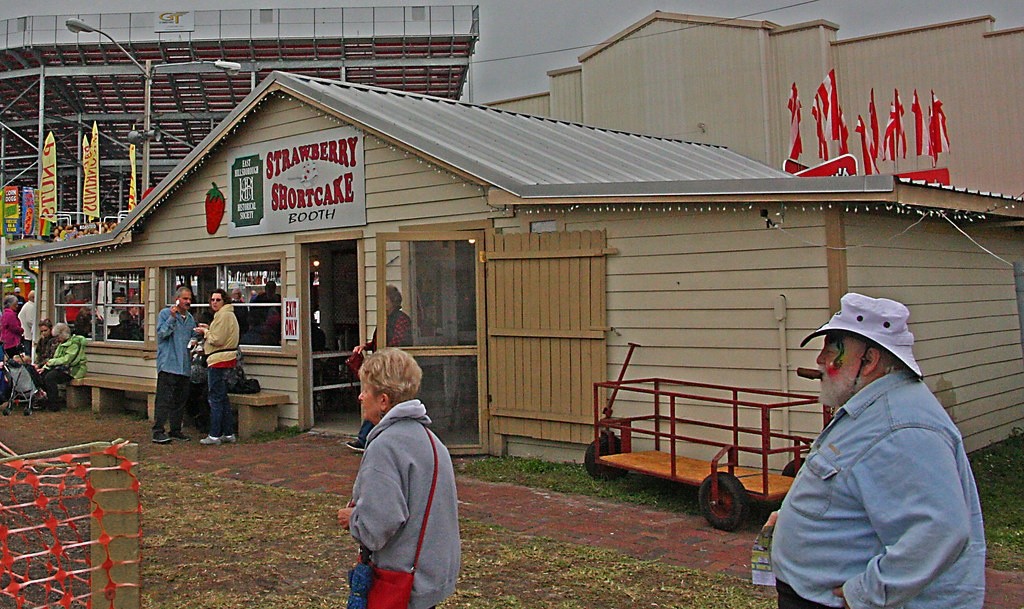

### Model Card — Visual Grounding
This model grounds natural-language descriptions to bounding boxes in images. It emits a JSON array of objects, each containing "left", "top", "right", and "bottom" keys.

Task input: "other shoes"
[
  {"left": 346, "top": 441, "right": 366, "bottom": 451},
  {"left": 32, "top": 398, "right": 46, "bottom": 410}
]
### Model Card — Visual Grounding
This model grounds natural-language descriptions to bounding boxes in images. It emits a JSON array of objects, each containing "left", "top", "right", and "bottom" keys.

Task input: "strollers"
[{"left": 1, "top": 343, "right": 48, "bottom": 416}]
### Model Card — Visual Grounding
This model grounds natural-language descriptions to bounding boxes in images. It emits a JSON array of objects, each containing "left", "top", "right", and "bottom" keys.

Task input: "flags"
[
  {"left": 911, "top": 89, "right": 950, "bottom": 167},
  {"left": 855, "top": 88, "right": 907, "bottom": 176},
  {"left": 787, "top": 82, "right": 802, "bottom": 160},
  {"left": 39, "top": 119, "right": 137, "bottom": 221},
  {"left": 811, "top": 69, "right": 849, "bottom": 161}
]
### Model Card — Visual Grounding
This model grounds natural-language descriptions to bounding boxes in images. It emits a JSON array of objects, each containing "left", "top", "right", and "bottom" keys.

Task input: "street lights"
[{"left": 65, "top": 17, "right": 241, "bottom": 202}]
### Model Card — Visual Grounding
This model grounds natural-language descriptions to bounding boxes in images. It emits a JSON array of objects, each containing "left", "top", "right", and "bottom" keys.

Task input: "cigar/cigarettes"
[{"left": 797, "top": 368, "right": 820, "bottom": 379}]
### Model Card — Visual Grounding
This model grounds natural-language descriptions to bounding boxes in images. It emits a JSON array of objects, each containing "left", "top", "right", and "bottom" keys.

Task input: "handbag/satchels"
[
  {"left": 358, "top": 556, "right": 414, "bottom": 609},
  {"left": 345, "top": 352, "right": 365, "bottom": 381},
  {"left": 55, "top": 364, "right": 70, "bottom": 374},
  {"left": 225, "top": 367, "right": 260, "bottom": 393},
  {"left": 198, "top": 348, "right": 237, "bottom": 368},
  {"left": 46, "top": 400, "right": 59, "bottom": 412}
]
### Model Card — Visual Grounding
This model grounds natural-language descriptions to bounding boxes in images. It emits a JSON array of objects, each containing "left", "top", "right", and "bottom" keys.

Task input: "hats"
[
  {"left": 801, "top": 292, "right": 923, "bottom": 378},
  {"left": 14, "top": 287, "right": 21, "bottom": 293}
]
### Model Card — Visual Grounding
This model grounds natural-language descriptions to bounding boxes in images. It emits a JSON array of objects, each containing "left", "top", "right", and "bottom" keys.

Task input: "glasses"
[{"left": 212, "top": 299, "right": 223, "bottom": 302}]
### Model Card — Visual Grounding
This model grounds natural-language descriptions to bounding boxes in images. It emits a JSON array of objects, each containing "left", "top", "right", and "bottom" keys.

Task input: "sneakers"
[
  {"left": 152, "top": 432, "right": 171, "bottom": 442},
  {"left": 167, "top": 432, "right": 190, "bottom": 441},
  {"left": 221, "top": 433, "right": 237, "bottom": 442},
  {"left": 199, "top": 434, "right": 221, "bottom": 444}
]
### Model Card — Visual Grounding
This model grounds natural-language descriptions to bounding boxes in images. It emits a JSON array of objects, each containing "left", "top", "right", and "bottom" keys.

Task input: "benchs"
[
  {"left": 58, "top": 377, "right": 93, "bottom": 408},
  {"left": 84, "top": 375, "right": 290, "bottom": 438}
]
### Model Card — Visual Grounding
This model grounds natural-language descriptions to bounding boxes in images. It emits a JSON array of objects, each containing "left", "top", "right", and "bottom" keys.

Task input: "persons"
[
  {"left": 338, "top": 347, "right": 460, "bottom": 609},
  {"left": 175, "top": 281, "right": 281, "bottom": 346},
  {"left": 193, "top": 289, "right": 239, "bottom": 445},
  {"left": 62, "top": 286, "right": 144, "bottom": 341},
  {"left": 152, "top": 287, "right": 202, "bottom": 444},
  {"left": 311, "top": 318, "right": 326, "bottom": 416},
  {"left": 345, "top": 284, "right": 413, "bottom": 451},
  {"left": 54, "top": 221, "right": 117, "bottom": 242},
  {"left": 770, "top": 293, "right": 986, "bottom": 609},
  {"left": 0, "top": 288, "right": 87, "bottom": 410}
]
